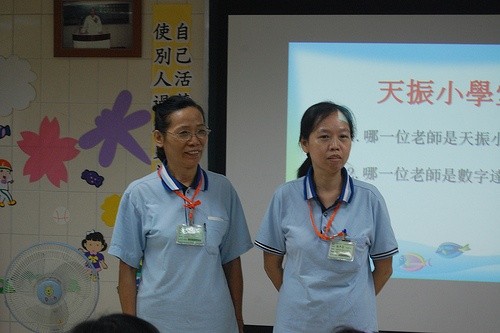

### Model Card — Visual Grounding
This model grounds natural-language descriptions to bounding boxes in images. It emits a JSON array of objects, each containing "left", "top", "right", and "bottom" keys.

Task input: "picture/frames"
[{"left": 54, "top": 0, "right": 143, "bottom": 58}]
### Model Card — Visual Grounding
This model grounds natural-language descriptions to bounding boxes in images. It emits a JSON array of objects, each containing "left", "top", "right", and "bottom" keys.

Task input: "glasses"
[{"left": 153, "top": 129, "right": 211, "bottom": 140}]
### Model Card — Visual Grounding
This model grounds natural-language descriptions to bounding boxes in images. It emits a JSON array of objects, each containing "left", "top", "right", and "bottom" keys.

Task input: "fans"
[{"left": 4, "top": 241, "right": 100, "bottom": 333}]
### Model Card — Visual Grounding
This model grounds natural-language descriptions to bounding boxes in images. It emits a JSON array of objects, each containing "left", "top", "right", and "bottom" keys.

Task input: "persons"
[
  {"left": 80, "top": 7, "right": 104, "bottom": 34},
  {"left": 107, "top": 96, "right": 254, "bottom": 333},
  {"left": 252, "top": 101, "right": 399, "bottom": 333}
]
